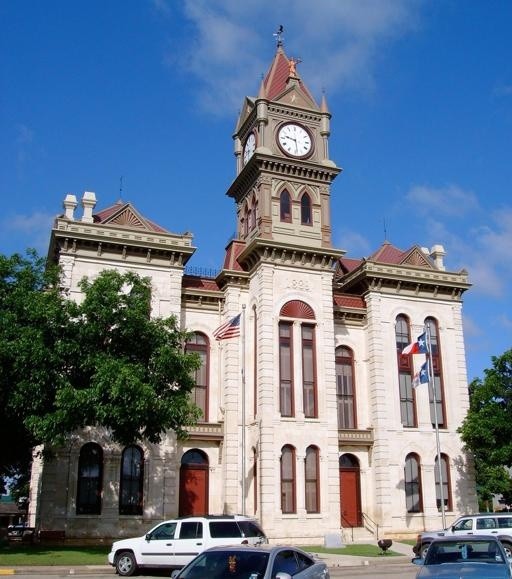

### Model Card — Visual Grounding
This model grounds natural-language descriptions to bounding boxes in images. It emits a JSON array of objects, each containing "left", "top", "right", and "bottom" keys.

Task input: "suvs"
[
  {"left": 108, "top": 514, "right": 269, "bottom": 577},
  {"left": 412, "top": 512, "right": 512, "bottom": 558}
]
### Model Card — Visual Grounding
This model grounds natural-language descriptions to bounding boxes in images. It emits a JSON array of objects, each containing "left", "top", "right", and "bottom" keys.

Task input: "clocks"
[
  {"left": 242, "top": 129, "right": 260, "bottom": 168},
  {"left": 274, "top": 119, "right": 315, "bottom": 160}
]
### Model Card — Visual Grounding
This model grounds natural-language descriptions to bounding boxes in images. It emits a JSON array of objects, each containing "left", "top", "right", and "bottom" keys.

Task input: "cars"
[
  {"left": 410, "top": 535, "right": 511, "bottom": 579},
  {"left": 171, "top": 543, "right": 331, "bottom": 579}
]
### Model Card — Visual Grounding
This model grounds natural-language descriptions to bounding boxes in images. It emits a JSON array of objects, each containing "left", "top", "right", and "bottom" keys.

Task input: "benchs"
[{"left": 37, "top": 530, "right": 67, "bottom": 546}]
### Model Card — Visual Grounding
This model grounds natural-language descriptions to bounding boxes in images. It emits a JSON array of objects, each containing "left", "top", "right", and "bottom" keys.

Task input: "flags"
[
  {"left": 411, "top": 361, "right": 430, "bottom": 389},
  {"left": 212, "top": 313, "right": 241, "bottom": 341},
  {"left": 401, "top": 332, "right": 430, "bottom": 355}
]
